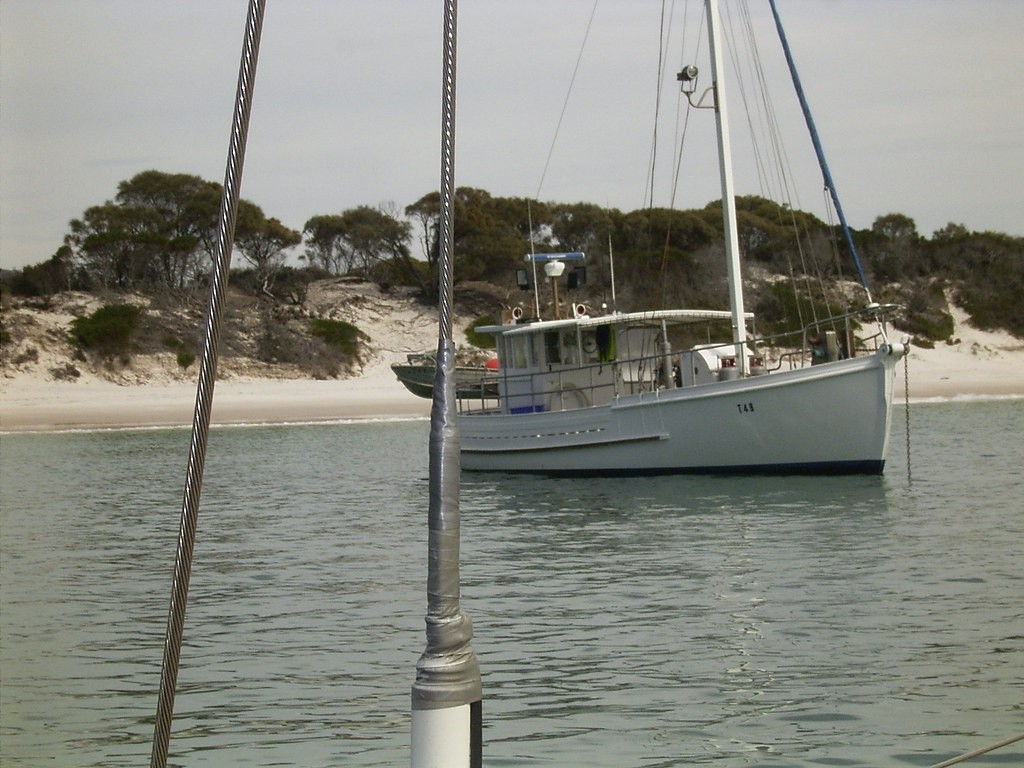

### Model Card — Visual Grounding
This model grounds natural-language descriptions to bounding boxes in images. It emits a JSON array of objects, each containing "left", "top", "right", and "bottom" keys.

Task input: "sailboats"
[{"left": 458, "top": 0, "right": 912, "bottom": 477}]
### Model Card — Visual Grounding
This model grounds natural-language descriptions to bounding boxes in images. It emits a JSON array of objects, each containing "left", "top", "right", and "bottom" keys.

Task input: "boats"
[{"left": 390, "top": 343, "right": 501, "bottom": 400}]
